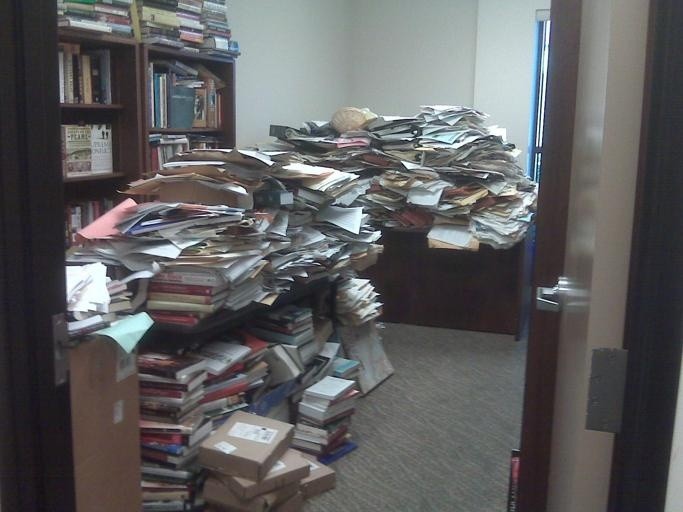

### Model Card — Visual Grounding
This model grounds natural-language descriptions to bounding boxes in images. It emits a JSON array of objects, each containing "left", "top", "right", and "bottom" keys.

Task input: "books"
[{"left": 58, "top": 1, "right": 390, "bottom": 510}]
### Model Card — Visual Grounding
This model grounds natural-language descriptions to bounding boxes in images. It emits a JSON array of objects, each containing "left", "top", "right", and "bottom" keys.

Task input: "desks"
[
  {"left": 139, "top": 271, "right": 335, "bottom": 346},
  {"left": 357, "top": 217, "right": 536, "bottom": 341}
]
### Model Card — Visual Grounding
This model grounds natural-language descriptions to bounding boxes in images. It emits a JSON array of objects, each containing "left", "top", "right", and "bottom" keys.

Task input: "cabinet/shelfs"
[
  {"left": 145, "top": 43, "right": 235, "bottom": 175},
  {"left": 58, "top": 26, "right": 143, "bottom": 185}
]
